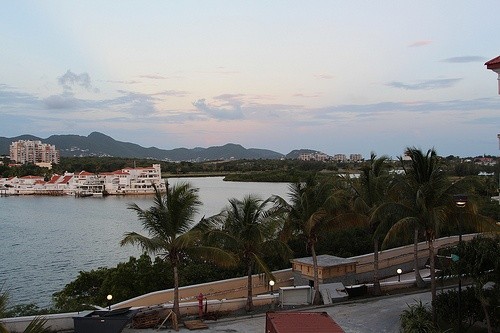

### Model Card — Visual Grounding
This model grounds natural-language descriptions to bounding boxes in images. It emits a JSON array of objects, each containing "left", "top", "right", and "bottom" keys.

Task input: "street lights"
[{"left": 455, "top": 195, "right": 466, "bottom": 331}]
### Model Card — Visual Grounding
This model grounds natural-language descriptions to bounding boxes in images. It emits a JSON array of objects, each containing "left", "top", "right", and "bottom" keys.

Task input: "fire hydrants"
[{"left": 197, "top": 293, "right": 205, "bottom": 317}]
